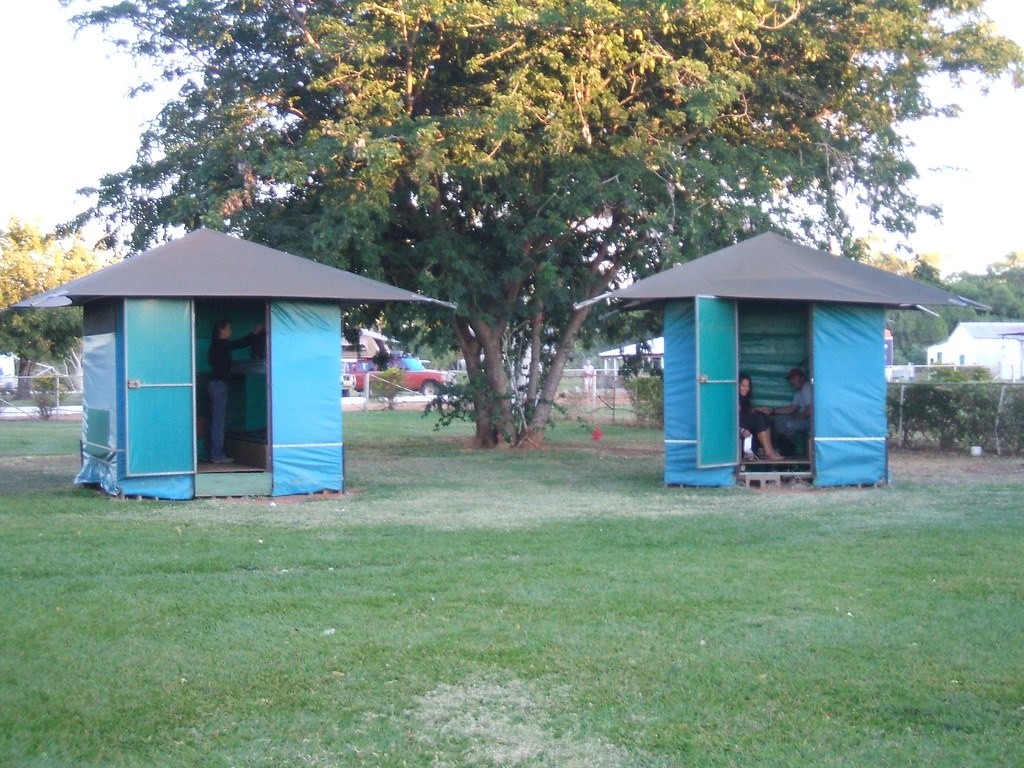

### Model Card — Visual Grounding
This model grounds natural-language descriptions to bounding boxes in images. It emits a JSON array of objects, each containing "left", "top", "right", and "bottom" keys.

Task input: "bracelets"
[
  {"left": 770, "top": 407, "right": 776, "bottom": 414},
  {"left": 755, "top": 407, "right": 757, "bottom": 413}
]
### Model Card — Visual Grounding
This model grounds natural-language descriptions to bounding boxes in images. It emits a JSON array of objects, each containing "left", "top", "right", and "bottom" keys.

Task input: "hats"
[{"left": 785, "top": 368, "right": 803, "bottom": 379}]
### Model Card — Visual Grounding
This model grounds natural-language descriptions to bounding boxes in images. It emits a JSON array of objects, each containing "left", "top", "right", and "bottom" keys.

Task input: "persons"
[
  {"left": 203, "top": 316, "right": 264, "bottom": 466},
  {"left": 738, "top": 366, "right": 813, "bottom": 460}
]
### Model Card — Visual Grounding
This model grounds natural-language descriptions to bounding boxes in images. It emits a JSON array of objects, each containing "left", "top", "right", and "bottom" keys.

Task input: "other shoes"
[
  {"left": 765, "top": 452, "right": 785, "bottom": 461},
  {"left": 211, "top": 458, "right": 234, "bottom": 463}
]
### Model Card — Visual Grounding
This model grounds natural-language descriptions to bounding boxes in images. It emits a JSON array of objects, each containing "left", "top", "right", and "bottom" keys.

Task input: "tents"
[
  {"left": 341, "top": 329, "right": 402, "bottom": 361},
  {"left": 598, "top": 336, "right": 664, "bottom": 379}
]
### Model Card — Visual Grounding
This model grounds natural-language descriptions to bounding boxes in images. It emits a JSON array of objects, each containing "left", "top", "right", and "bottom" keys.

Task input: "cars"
[{"left": 342, "top": 357, "right": 445, "bottom": 397}]
